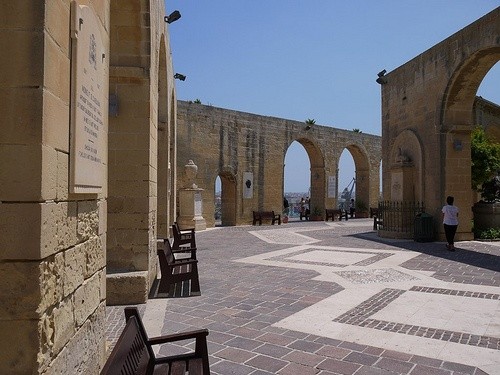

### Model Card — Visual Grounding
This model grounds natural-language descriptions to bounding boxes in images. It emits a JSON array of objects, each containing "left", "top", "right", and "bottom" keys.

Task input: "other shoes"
[{"left": 444, "top": 242, "right": 455, "bottom": 251}]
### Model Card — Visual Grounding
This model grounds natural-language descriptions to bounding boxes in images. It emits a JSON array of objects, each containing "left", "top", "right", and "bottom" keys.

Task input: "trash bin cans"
[{"left": 415, "top": 212, "right": 433, "bottom": 243}]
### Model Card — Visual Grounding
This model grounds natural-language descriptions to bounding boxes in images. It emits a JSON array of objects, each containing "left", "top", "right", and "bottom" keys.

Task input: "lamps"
[
  {"left": 305, "top": 125, "right": 311, "bottom": 130},
  {"left": 173, "top": 73, "right": 186, "bottom": 81},
  {"left": 164, "top": 10, "right": 181, "bottom": 24},
  {"left": 453, "top": 140, "right": 463, "bottom": 150},
  {"left": 376, "top": 69, "right": 387, "bottom": 85}
]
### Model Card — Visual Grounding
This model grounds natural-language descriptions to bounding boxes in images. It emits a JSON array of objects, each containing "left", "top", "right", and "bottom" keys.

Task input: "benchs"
[
  {"left": 370, "top": 208, "right": 378, "bottom": 218},
  {"left": 157, "top": 238, "right": 201, "bottom": 297},
  {"left": 172, "top": 222, "right": 196, "bottom": 249},
  {"left": 325, "top": 209, "right": 348, "bottom": 221},
  {"left": 300, "top": 208, "right": 309, "bottom": 221},
  {"left": 100, "top": 307, "right": 211, "bottom": 375},
  {"left": 253, "top": 211, "right": 281, "bottom": 226}
]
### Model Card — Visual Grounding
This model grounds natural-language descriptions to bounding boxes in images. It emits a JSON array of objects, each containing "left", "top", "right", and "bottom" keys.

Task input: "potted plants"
[
  {"left": 355, "top": 202, "right": 369, "bottom": 218},
  {"left": 471, "top": 124, "right": 500, "bottom": 241},
  {"left": 282, "top": 208, "right": 289, "bottom": 223}
]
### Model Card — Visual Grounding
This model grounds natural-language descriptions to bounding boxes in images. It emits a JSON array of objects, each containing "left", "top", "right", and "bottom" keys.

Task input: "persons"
[
  {"left": 441, "top": 195, "right": 460, "bottom": 253},
  {"left": 299, "top": 196, "right": 310, "bottom": 222},
  {"left": 339, "top": 197, "right": 356, "bottom": 221},
  {"left": 283, "top": 197, "right": 289, "bottom": 217}
]
